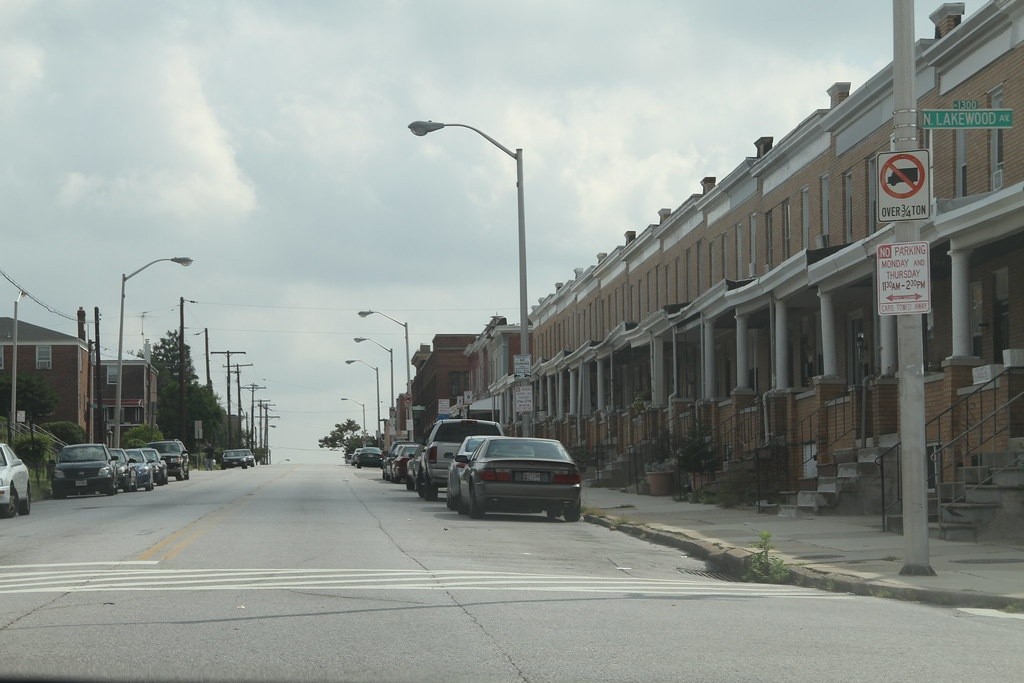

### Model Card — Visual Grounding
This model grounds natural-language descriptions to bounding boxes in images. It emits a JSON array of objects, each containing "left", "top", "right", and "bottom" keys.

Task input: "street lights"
[
  {"left": 358, "top": 310, "right": 413, "bottom": 442},
  {"left": 341, "top": 398, "right": 366, "bottom": 447},
  {"left": 354, "top": 336, "right": 395, "bottom": 444},
  {"left": 10, "top": 291, "right": 27, "bottom": 453},
  {"left": 114, "top": 256, "right": 193, "bottom": 449},
  {"left": 408, "top": 120, "right": 530, "bottom": 438},
  {"left": 345, "top": 360, "right": 380, "bottom": 449}
]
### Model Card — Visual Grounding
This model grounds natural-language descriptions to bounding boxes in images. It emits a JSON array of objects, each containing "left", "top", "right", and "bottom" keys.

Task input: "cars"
[
  {"left": 345, "top": 448, "right": 385, "bottom": 469},
  {"left": 221, "top": 449, "right": 256, "bottom": 470},
  {"left": 0, "top": 443, "right": 31, "bottom": 517},
  {"left": 381, "top": 441, "right": 426, "bottom": 484},
  {"left": 52, "top": 443, "right": 118, "bottom": 499},
  {"left": 107, "top": 448, "right": 168, "bottom": 492},
  {"left": 455, "top": 438, "right": 582, "bottom": 522},
  {"left": 447, "top": 435, "right": 536, "bottom": 511}
]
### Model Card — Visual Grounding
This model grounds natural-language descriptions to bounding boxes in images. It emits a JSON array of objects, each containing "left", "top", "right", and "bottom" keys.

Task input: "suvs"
[
  {"left": 407, "top": 418, "right": 506, "bottom": 501},
  {"left": 144, "top": 439, "right": 190, "bottom": 480}
]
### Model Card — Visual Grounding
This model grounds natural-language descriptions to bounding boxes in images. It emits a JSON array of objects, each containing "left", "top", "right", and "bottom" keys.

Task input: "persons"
[{"left": 203, "top": 441, "right": 215, "bottom": 471}]
